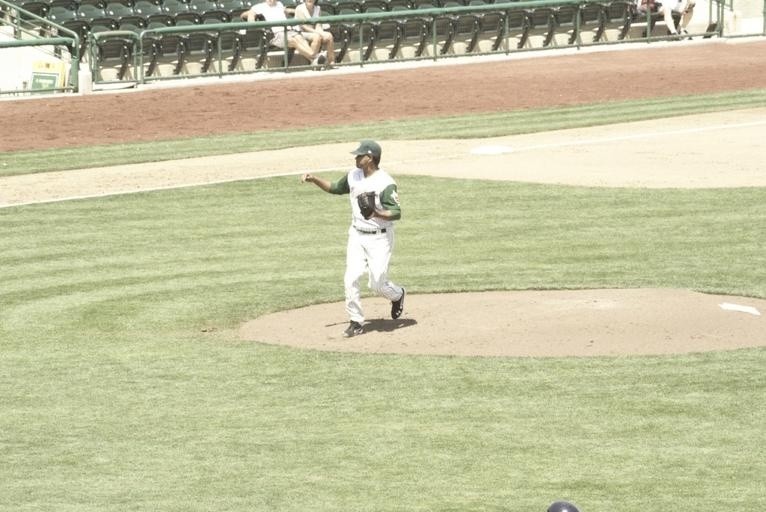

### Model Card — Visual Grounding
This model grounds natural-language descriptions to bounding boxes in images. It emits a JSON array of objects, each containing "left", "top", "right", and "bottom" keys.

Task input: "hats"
[{"left": 349, "top": 139, "right": 381, "bottom": 157}]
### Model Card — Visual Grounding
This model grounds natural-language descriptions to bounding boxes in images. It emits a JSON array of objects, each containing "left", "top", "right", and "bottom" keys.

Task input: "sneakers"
[
  {"left": 669, "top": 30, "right": 693, "bottom": 42},
  {"left": 342, "top": 320, "right": 364, "bottom": 337},
  {"left": 312, "top": 50, "right": 328, "bottom": 69},
  {"left": 391, "top": 287, "right": 404, "bottom": 319}
]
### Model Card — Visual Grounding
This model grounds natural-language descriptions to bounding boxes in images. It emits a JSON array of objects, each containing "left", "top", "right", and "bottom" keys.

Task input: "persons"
[
  {"left": 291, "top": 0, "right": 340, "bottom": 70},
  {"left": 245, "top": 0, "right": 325, "bottom": 71},
  {"left": 297, "top": 138, "right": 404, "bottom": 338},
  {"left": 638, "top": 0, "right": 694, "bottom": 41}
]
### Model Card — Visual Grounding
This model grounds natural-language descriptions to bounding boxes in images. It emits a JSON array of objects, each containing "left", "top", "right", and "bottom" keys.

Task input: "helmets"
[{"left": 546, "top": 502, "right": 579, "bottom": 512}]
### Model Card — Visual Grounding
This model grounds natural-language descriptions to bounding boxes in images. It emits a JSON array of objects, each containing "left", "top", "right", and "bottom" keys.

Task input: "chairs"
[{"left": 0, "top": 2, "right": 692, "bottom": 87}]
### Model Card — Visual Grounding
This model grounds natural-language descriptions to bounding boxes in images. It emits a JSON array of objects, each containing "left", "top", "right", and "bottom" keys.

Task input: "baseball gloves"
[{"left": 358, "top": 192, "right": 376, "bottom": 219}]
